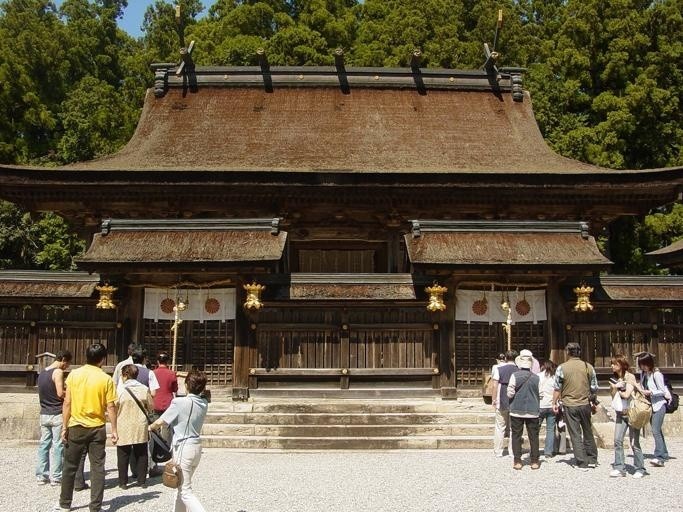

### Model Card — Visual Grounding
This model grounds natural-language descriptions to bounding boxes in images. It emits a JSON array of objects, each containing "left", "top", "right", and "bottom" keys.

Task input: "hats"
[
  {"left": 148, "top": 428, "right": 174, "bottom": 465},
  {"left": 514, "top": 349, "right": 533, "bottom": 369}
]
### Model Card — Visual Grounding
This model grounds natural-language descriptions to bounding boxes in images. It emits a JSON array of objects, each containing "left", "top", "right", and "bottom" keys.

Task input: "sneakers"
[
  {"left": 531, "top": 461, "right": 541, "bottom": 469},
  {"left": 36, "top": 479, "right": 49, "bottom": 485},
  {"left": 588, "top": 463, "right": 597, "bottom": 468},
  {"left": 572, "top": 462, "right": 589, "bottom": 471},
  {"left": 119, "top": 484, "right": 127, "bottom": 489},
  {"left": 513, "top": 462, "right": 523, "bottom": 470},
  {"left": 650, "top": 453, "right": 669, "bottom": 466},
  {"left": 544, "top": 452, "right": 552, "bottom": 459},
  {"left": 493, "top": 451, "right": 514, "bottom": 457},
  {"left": 632, "top": 471, "right": 644, "bottom": 479},
  {"left": 132, "top": 473, "right": 145, "bottom": 486},
  {"left": 54, "top": 496, "right": 70, "bottom": 512},
  {"left": 608, "top": 469, "right": 627, "bottom": 478},
  {"left": 75, "top": 487, "right": 81, "bottom": 491},
  {"left": 50, "top": 480, "right": 62, "bottom": 486}
]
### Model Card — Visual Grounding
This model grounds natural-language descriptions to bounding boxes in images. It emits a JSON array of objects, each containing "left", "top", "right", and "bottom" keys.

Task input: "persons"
[
  {"left": 636, "top": 352, "right": 672, "bottom": 467},
  {"left": 110, "top": 341, "right": 147, "bottom": 385},
  {"left": 72, "top": 448, "right": 87, "bottom": 492},
  {"left": 488, "top": 340, "right": 598, "bottom": 469},
  {"left": 36, "top": 349, "right": 71, "bottom": 488},
  {"left": 112, "top": 363, "right": 154, "bottom": 491},
  {"left": 150, "top": 354, "right": 178, "bottom": 460},
  {"left": 608, "top": 354, "right": 648, "bottom": 480},
  {"left": 58, "top": 342, "right": 119, "bottom": 512},
  {"left": 125, "top": 347, "right": 160, "bottom": 399},
  {"left": 147, "top": 368, "right": 211, "bottom": 512}
]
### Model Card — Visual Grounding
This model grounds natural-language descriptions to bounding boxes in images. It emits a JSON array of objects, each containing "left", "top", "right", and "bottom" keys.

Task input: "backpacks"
[
  {"left": 663, "top": 377, "right": 679, "bottom": 413},
  {"left": 626, "top": 394, "right": 654, "bottom": 430}
]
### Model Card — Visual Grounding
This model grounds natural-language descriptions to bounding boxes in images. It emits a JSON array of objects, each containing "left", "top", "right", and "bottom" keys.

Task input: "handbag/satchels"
[{"left": 162, "top": 462, "right": 184, "bottom": 490}]
